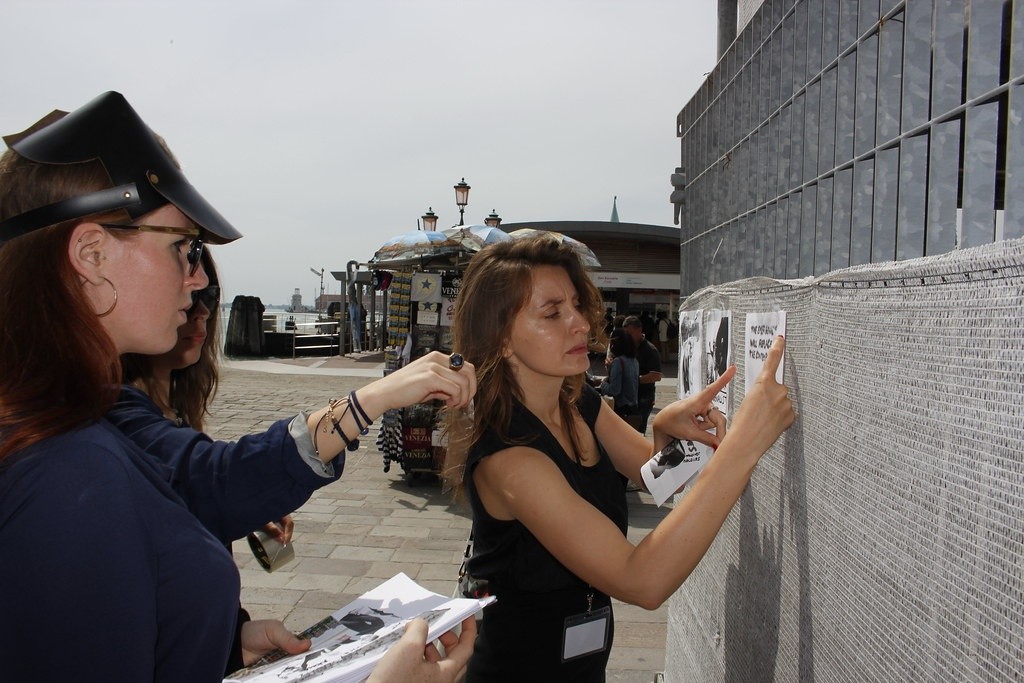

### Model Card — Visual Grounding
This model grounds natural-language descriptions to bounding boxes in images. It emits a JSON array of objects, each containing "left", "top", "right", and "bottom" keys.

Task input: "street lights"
[{"left": 416, "top": 176, "right": 503, "bottom": 232}]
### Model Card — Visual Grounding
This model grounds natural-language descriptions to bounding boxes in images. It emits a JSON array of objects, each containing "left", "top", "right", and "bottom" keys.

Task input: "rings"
[
  {"left": 449, "top": 353, "right": 464, "bottom": 370},
  {"left": 706, "top": 406, "right": 719, "bottom": 415}
]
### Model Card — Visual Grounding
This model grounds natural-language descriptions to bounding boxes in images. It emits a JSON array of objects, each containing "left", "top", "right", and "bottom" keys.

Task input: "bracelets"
[{"left": 313, "top": 390, "right": 375, "bottom": 458}]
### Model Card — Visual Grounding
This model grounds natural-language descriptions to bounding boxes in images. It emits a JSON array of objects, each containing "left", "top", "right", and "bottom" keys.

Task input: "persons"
[
  {"left": 120, "top": 241, "right": 479, "bottom": 558},
  {"left": 443, "top": 230, "right": 800, "bottom": 683},
  {"left": 599, "top": 304, "right": 674, "bottom": 493},
  {"left": 0, "top": 90, "right": 474, "bottom": 683}
]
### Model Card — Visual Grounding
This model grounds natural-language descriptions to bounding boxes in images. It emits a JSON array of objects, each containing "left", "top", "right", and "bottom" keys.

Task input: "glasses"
[{"left": 96, "top": 223, "right": 205, "bottom": 278}]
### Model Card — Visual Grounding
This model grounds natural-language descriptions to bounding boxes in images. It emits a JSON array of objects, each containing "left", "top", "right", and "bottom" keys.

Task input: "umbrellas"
[{"left": 368, "top": 224, "right": 602, "bottom": 269}]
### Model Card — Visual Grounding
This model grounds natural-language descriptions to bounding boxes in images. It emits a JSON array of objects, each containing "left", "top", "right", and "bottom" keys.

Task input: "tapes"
[{"left": 245, "top": 526, "right": 294, "bottom": 573}]
